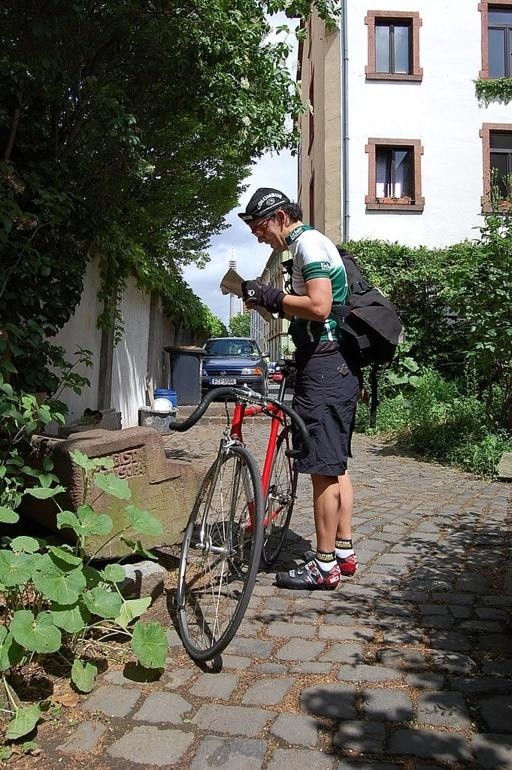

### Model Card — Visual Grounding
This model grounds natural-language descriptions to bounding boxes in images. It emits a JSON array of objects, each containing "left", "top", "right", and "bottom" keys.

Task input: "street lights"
[{"left": 240, "top": 322, "right": 268, "bottom": 354}]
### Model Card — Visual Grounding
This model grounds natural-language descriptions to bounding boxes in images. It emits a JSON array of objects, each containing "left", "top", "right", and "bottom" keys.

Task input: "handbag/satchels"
[{"left": 335, "top": 242, "right": 406, "bottom": 372}]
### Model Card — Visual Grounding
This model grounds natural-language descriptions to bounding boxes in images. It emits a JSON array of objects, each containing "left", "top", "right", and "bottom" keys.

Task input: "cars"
[{"left": 266, "top": 360, "right": 286, "bottom": 383}]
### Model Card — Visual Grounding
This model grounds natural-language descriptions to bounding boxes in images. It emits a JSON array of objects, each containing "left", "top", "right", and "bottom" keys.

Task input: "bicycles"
[{"left": 169, "top": 359, "right": 312, "bottom": 662}]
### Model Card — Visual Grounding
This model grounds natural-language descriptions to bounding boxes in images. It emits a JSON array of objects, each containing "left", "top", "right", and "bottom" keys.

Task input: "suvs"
[{"left": 202, "top": 335, "right": 270, "bottom": 401}]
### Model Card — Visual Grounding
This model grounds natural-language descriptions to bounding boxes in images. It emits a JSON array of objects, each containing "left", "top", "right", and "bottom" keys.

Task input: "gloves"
[{"left": 241, "top": 279, "right": 285, "bottom": 314}]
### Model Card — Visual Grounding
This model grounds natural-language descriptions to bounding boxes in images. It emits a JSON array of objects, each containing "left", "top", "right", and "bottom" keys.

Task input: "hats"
[{"left": 237, "top": 186, "right": 291, "bottom": 225}]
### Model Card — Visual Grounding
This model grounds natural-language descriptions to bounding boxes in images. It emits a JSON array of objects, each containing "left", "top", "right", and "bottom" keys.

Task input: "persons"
[{"left": 241, "top": 188, "right": 363, "bottom": 590}]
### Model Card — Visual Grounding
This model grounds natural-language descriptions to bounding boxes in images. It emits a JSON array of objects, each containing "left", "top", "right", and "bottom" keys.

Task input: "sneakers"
[
  {"left": 275, "top": 558, "right": 342, "bottom": 591},
  {"left": 336, "top": 552, "right": 358, "bottom": 576}
]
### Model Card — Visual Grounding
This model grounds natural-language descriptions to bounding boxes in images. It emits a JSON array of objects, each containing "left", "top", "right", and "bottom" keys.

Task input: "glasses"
[{"left": 250, "top": 217, "right": 274, "bottom": 239}]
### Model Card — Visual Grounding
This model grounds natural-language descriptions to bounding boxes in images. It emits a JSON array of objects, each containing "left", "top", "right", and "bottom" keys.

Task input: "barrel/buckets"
[
  {"left": 154, "top": 387, "right": 179, "bottom": 409},
  {"left": 153, "top": 398, "right": 173, "bottom": 411}
]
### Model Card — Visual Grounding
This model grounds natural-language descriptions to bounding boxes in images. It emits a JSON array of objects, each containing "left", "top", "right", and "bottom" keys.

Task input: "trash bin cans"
[{"left": 163, "top": 345, "right": 206, "bottom": 406}]
